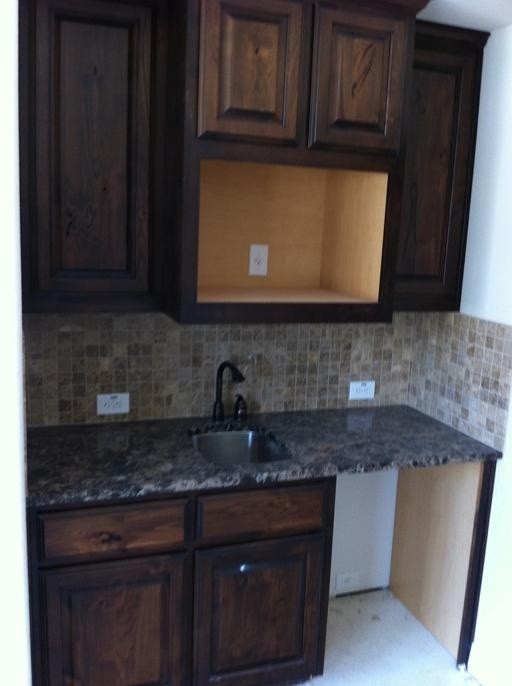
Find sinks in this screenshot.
[192,431,255,465]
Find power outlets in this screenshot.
[96,392,131,415]
[248,244,269,277]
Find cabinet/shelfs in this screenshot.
[189,481,325,686]
[157,0,412,150]
[157,142,398,324]
[394,40,481,304]
[32,0,156,304]
[38,494,192,686]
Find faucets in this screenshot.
[214,360,244,421]
